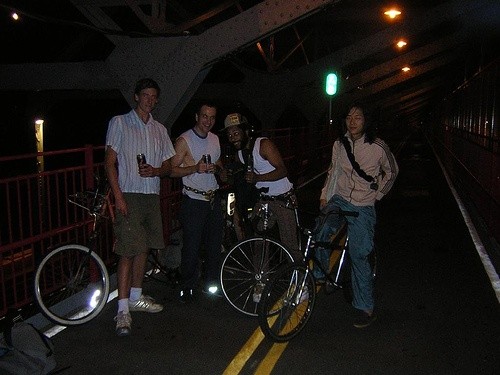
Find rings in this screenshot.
[247,178,249,180]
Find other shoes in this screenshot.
[201,281,224,297]
[179,287,193,304]
[305,277,326,285]
[352,309,377,328]
[253,283,270,303]
[300,290,309,304]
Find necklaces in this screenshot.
[352,136,356,140]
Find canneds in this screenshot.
[203,154,212,174]
[246,166,252,173]
[137,153,146,169]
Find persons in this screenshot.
[314,102,400,328]
[220,113,306,265]
[105,78,175,336]
[168,102,225,306]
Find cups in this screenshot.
[246,159,253,172]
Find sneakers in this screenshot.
[128,295,163,313]
[114,310,132,336]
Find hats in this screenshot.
[225,113,243,128]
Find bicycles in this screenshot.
[32,176,183,328]
[215,192,313,319]
[257,203,378,344]
